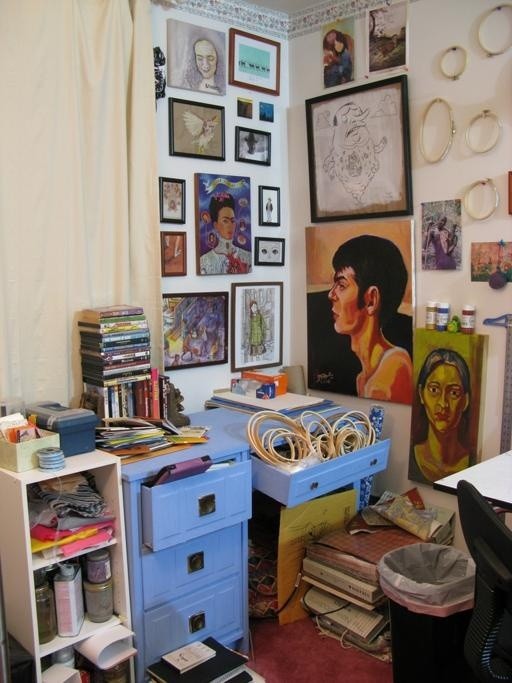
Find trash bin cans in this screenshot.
[377,544,478,682]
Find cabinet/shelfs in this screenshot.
[0,450,135,683]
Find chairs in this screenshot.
[456,479,512,683]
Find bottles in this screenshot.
[32,580,58,644]
[53,562,85,637]
[50,646,76,671]
[425,301,477,334]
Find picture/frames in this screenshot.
[305,74,413,224]
[160,290,229,372]
[254,237,285,266]
[159,230,187,278]
[234,126,272,166]
[158,177,187,224]
[231,281,283,372]
[228,27,283,97]
[167,97,226,161]
[258,185,281,226]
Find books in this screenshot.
[78,304,171,426]
[146,637,253,683]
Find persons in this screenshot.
[249,301,268,356]
[328,234,414,404]
[182,29,225,94]
[409,347,476,486]
[323,31,353,88]
[200,192,252,273]
[265,198,274,222]
[422,216,458,269]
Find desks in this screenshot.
[119,392,390,683]
[433,449,512,511]
